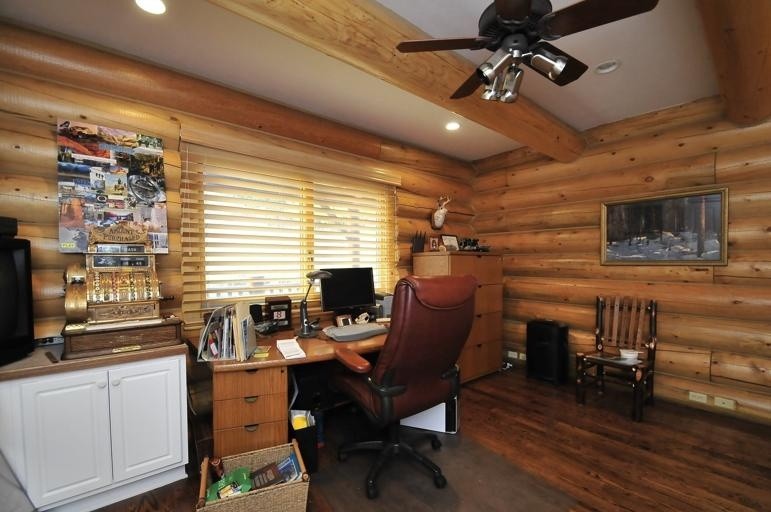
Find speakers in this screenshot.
[1,217,34,367]
[266,296,292,332]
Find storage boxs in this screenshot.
[412,251,503,385]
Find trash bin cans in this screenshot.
[289,422,318,472]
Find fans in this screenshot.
[396,0,659,100]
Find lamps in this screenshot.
[476,1,569,103]
[63,262,87,322]
[293,270,331,340]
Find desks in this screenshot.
[187,316,389,466]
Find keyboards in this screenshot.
[327,323,389,341]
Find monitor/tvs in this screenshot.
[319,267,376,312]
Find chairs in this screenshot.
[327,273,477,499]
[576,295,658,422]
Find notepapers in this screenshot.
[254,352,270,358]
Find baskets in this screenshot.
[195,439,310,512]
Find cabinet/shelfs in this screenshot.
[0,341,189,512]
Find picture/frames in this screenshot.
[600,185,728,266]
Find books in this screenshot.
[254,346,271,358]
